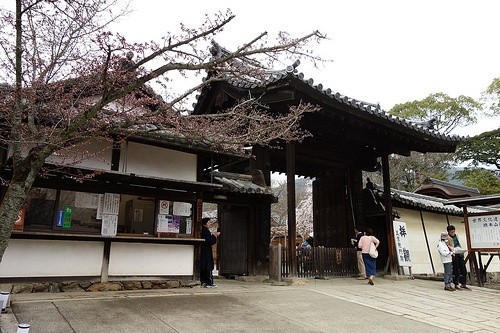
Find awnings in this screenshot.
[6,152,223,195]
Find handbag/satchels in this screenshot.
[369,241,379,259]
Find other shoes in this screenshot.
[454,286,460,290]
[368,277,374,285]
[444,287,452,290]
[357,277,365,280]
[200,282,217,288]
[460,286,469,290]
[450,286,456,291]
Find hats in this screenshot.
[440,232,449,240]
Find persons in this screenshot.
[447,225,470,290]
[437,233,455,291]
[200,217,220,287]
[351,225,368,279]
[358,226,380,285]
[296,234,313,275]
